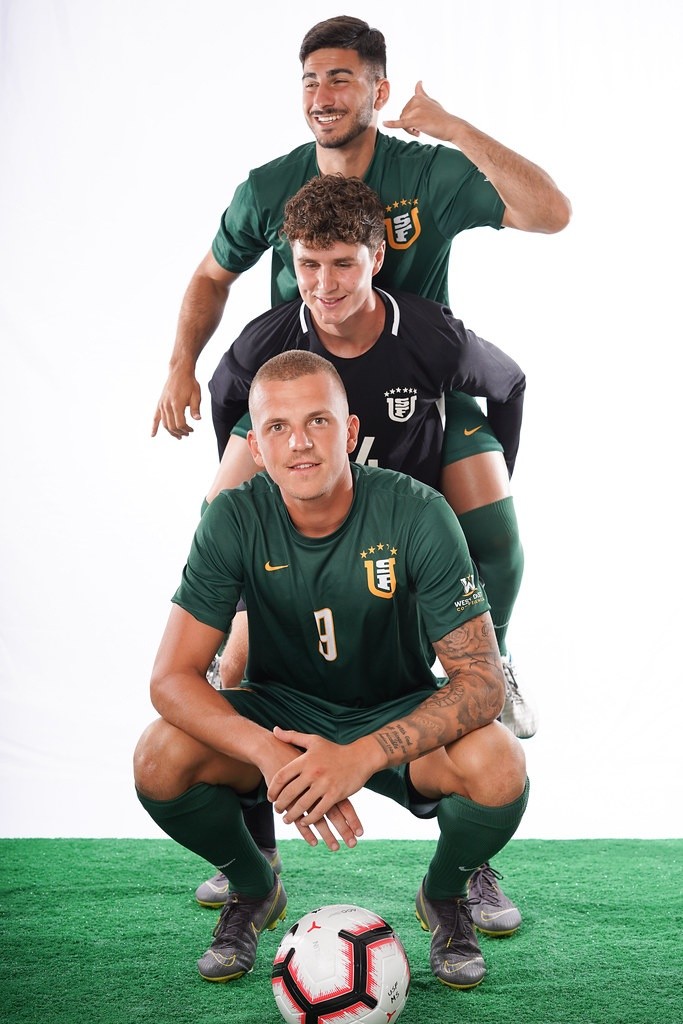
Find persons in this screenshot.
[193,170,525,937]
[135,350,531,990]
[150,14,574,738]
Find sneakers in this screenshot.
[195,847,282,907]
[497,653,538,739]
[466,859,521,937]
[197,870,286,981]
[414,872,486,990]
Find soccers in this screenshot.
[272,905,409,1024]
